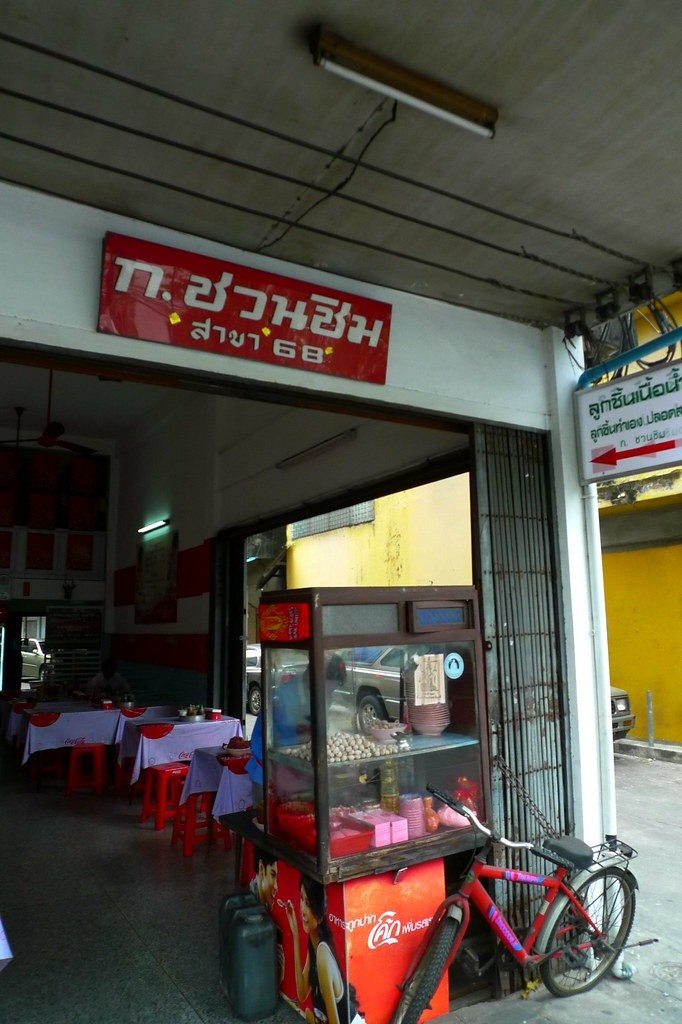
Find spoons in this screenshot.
[277,899,290,908]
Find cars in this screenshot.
[332,642,636,754]
[18,635,53,682]
[246,641,311,716]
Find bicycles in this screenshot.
[385,785,659,1024]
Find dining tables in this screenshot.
[6,697,96,765]
[21,701,185,765]
[126,713,244,785]
[192,746,253,828]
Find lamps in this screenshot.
[136,519,170,534]
[307,28,499,140]
[275,428,357,470]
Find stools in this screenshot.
[65,743,108,800]
[114,743,143,801]
[171,781,232,856]
[139,761,190,831]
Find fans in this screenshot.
[0,361,100,458]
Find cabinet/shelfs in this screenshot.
[255,587,494,883]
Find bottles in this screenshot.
[378,757,402,815]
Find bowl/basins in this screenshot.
[118,700,136,707]
[403,668,452,735]
[180,713,208,722]
[370,722,408,743]
[223,744,252,756]
